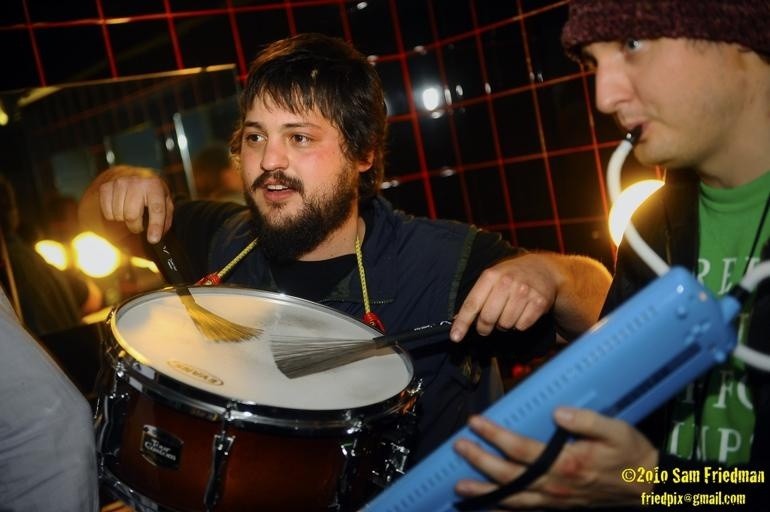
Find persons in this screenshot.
[452,3,769,512]
[77,34,614,512]
[1,172,82,337]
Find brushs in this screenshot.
[271,316,475,379]
[143,208,264,344]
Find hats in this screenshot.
[562,1,768,59]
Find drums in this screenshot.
[94,282,426,510]
